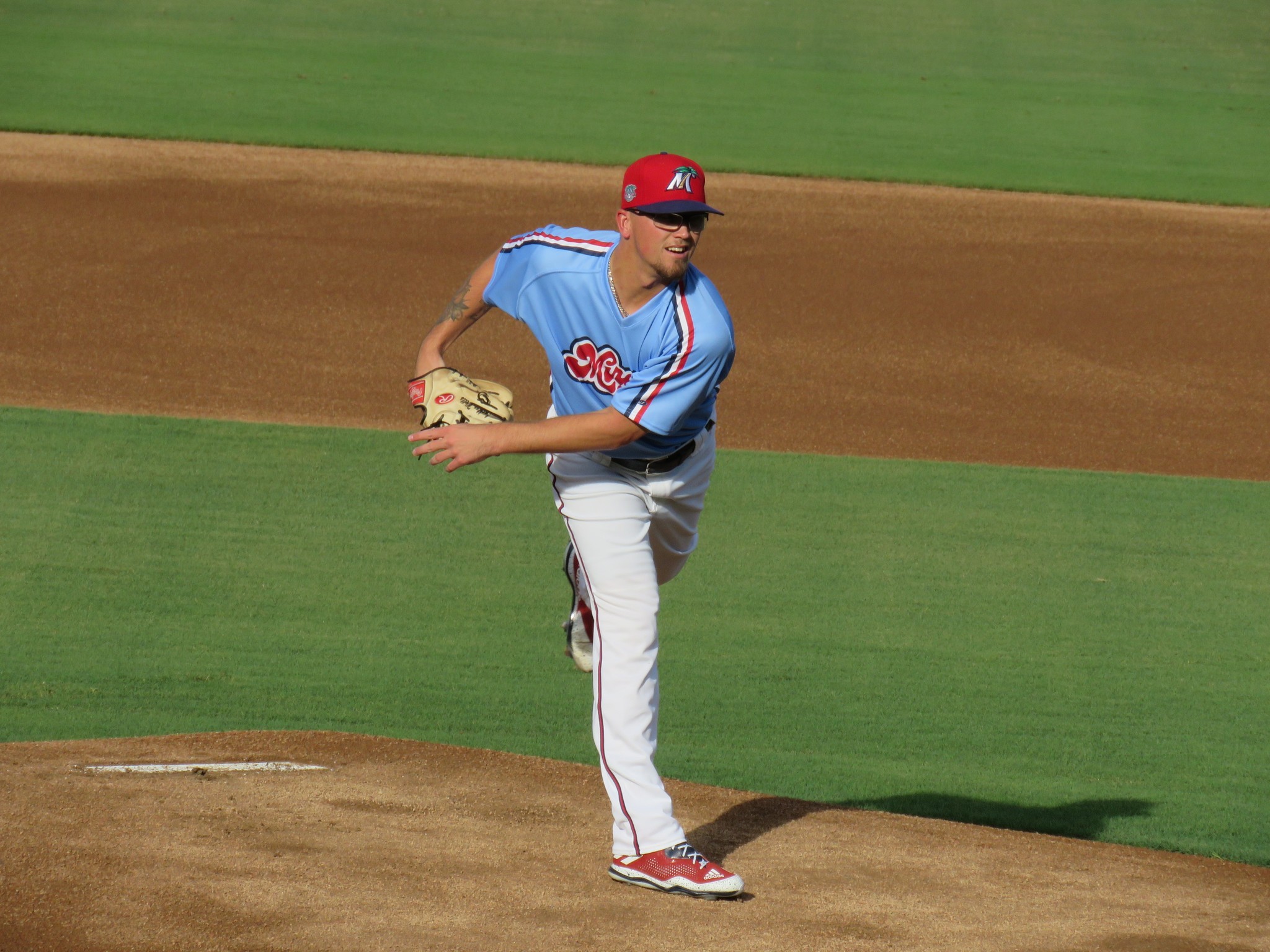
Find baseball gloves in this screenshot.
[408,366,515,441]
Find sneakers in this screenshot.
[561,540,595,673]
[608,842,744,900]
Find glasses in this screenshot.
[624,207,709,233]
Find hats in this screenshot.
[621,152,725,216]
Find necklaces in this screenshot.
[608,255,630,318]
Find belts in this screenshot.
[611,419,714,475]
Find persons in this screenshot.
[408,152,745,900]
